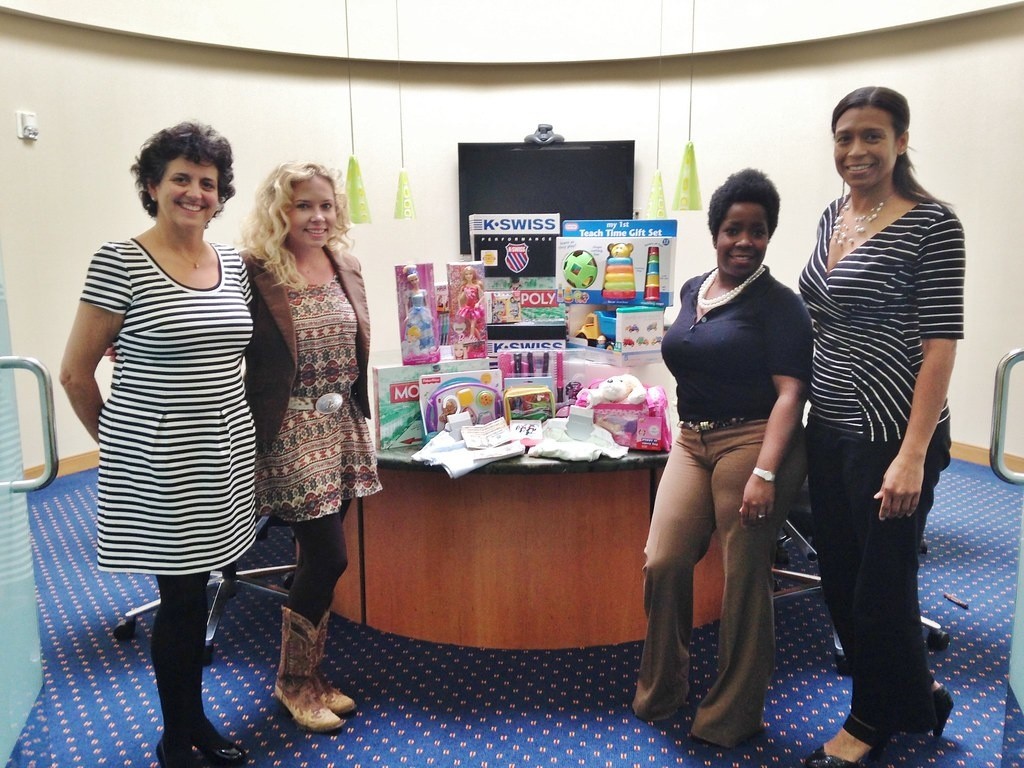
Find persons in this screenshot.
[59,123,259,768]
[402,265,438,353]
[458,267,483,344]
[799,86,966,768]
[633,170,814,746]
[105,161,385,730]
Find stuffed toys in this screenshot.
[581,374,647,408]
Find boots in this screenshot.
[275,605,357,733]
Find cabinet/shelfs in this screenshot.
[270,426,728,648]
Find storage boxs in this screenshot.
[578,377,671,453]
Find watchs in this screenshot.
[753,467,776,482]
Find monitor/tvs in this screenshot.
[458,140,635,254]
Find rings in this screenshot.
[757,515,767,519]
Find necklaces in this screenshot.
[833,191,896,245]
[155,227,205,269]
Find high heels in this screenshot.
[805,733,892,768]
[156,719,245,768]
[896,683,954,736]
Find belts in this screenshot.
[288,390,350,415]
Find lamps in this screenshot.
[343,0,704,223]
